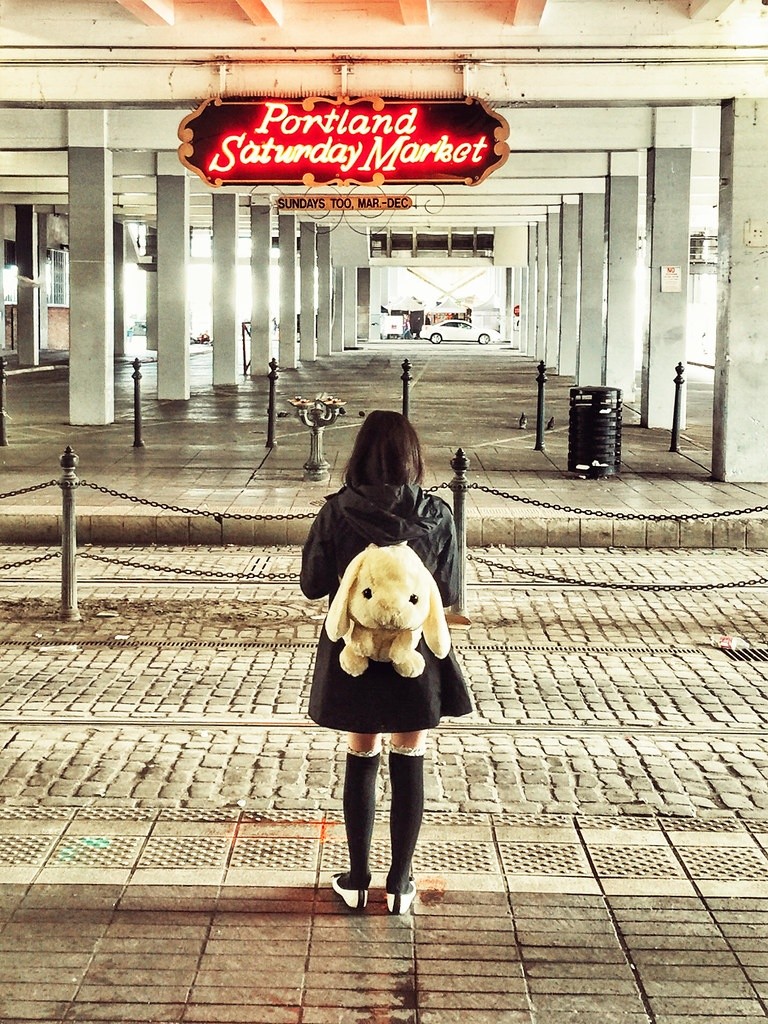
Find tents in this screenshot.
[431,297,466,323]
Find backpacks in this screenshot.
[326,538,453,679]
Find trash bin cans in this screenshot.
[565,385,624,481]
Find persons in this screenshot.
[299,410,474,913]
[124,315,278,343]
[402,315,471,339]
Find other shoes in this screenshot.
[333,873,370,910]
[387,878,416,915]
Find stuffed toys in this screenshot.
[324,540,451,678]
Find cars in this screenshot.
[419,321,502,345]
